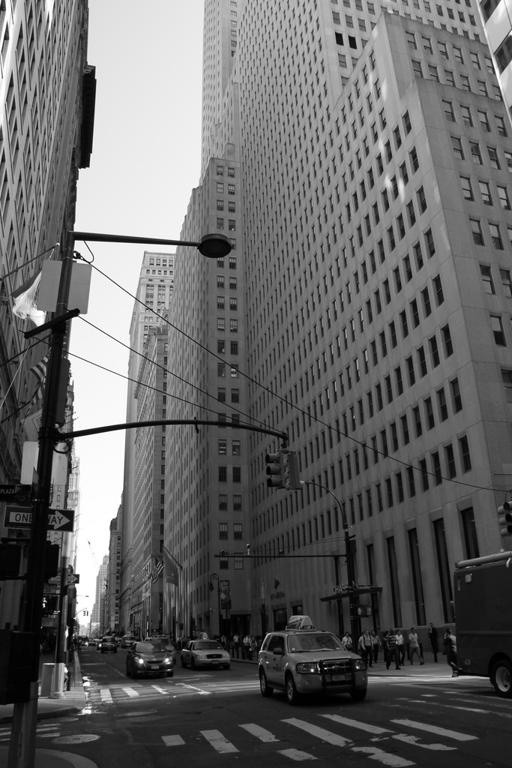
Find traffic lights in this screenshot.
[497,502,512,537]
[265,451,284,488]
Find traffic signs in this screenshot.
[4,506,74,533]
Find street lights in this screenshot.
[11,230,232,763]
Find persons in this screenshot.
[217,629,270,660]
[440,627,463,677]
[426,622,441,663]
[342,626,424,670]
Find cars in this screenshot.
[78,635,134,654]
[126,642,174,680]
[180,640,230,671]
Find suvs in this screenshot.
[257,630,367,704]
[144,634,177,665]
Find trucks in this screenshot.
[450,552,511,697]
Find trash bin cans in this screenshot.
[41,662,56,696]
